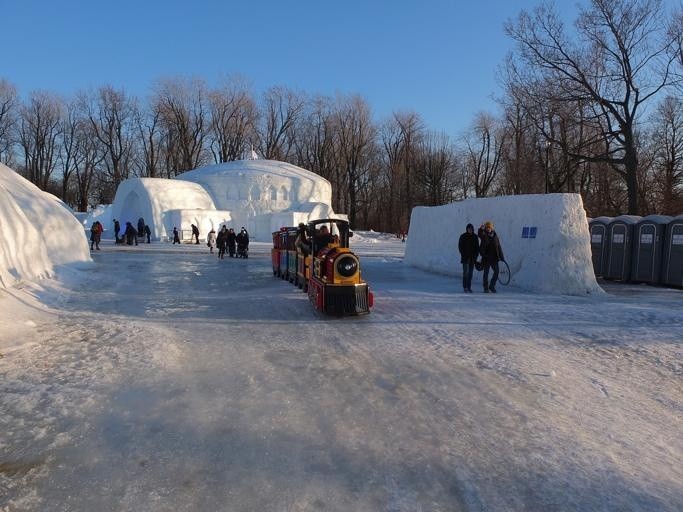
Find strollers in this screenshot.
[236,244,248,259]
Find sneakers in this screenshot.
[464,287,496,294]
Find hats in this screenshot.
[486,222,493,228]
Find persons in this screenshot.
[458,223,480,293]
[294,220,350,257]
[478,221,504,292]
[90,217,250,259]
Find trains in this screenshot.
[272,218,374,318]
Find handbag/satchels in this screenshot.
[475,262,483,271]
